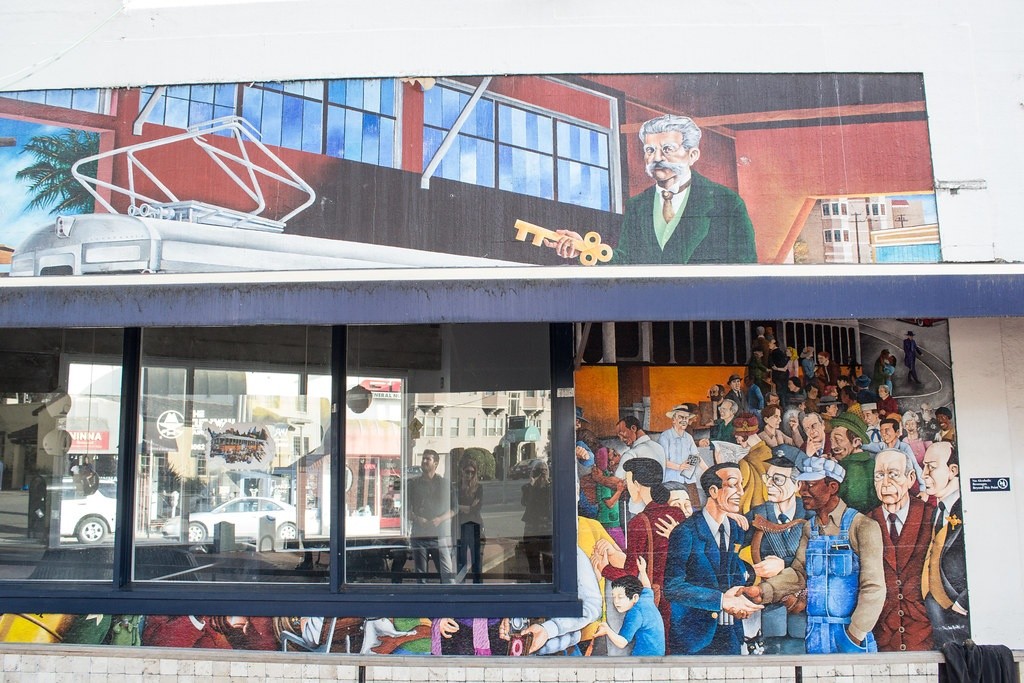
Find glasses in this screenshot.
[464,470,475,474]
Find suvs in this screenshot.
[59,476,116,545]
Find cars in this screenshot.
[159,496,320,542]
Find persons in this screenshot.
[520,461,554,586]
[163,488,179,517]
[450,460,484,584]
[247,483,259,507]
[71,463,81,483]
[406,449,453,583]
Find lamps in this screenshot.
[72,329,100,496]
[345,325,375,413]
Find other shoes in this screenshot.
[295,562,314,570]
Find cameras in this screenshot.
[532,470,542,478]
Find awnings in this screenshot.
[509,426,541,442]
